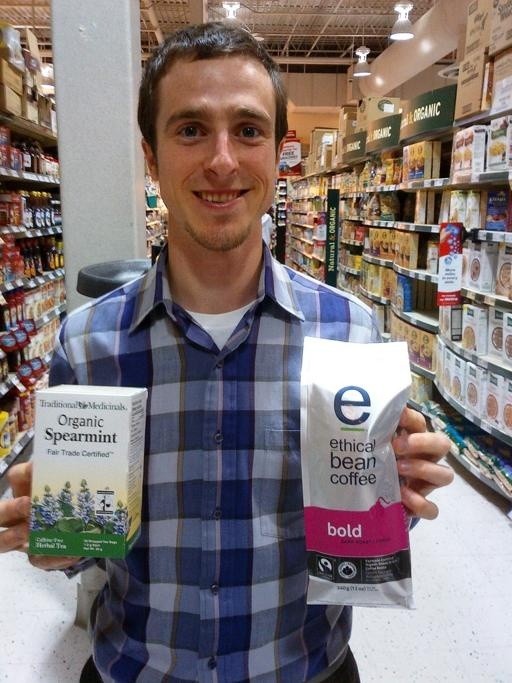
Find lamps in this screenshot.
[251,32,264,41]
[222,1,241,20]
[389,2,415,40]
[353,19,372,78]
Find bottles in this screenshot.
[0,290,34,454]
[0,141,63,284]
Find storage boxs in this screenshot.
[0,26,57,138]
[454,1,512,124]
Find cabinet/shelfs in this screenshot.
[145,174,169,259]
[275,177,286,263]
[0,109,67,478]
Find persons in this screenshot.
[0,20,454,683]
